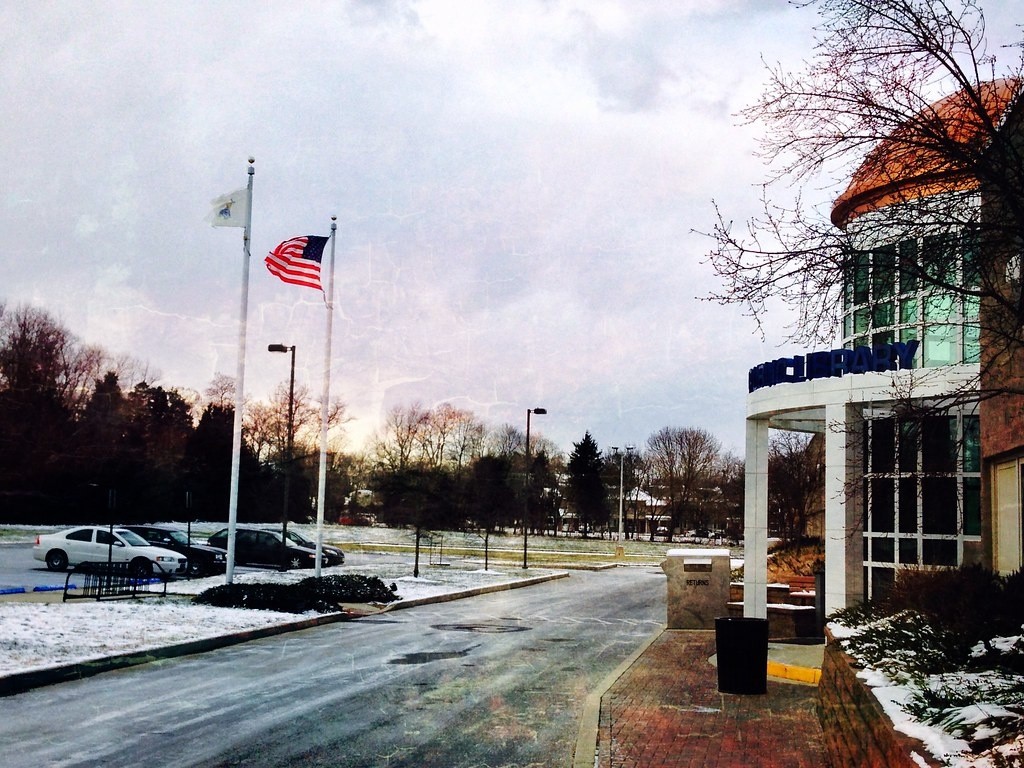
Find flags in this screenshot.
[264,235,329,290]
[205,188,252,227]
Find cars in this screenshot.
[120,525,236,578]
[687,529,712,537]
[562,524,591,532]
[33,527,188,580]
[208,527,329,573]
[258,528,345,568]
[656,526,669,536]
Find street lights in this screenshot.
[612,445,634,541]
[267,344,297,573]
[522,407,547,568]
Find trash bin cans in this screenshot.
[715,617,770,695]
[661,548,729,629]
